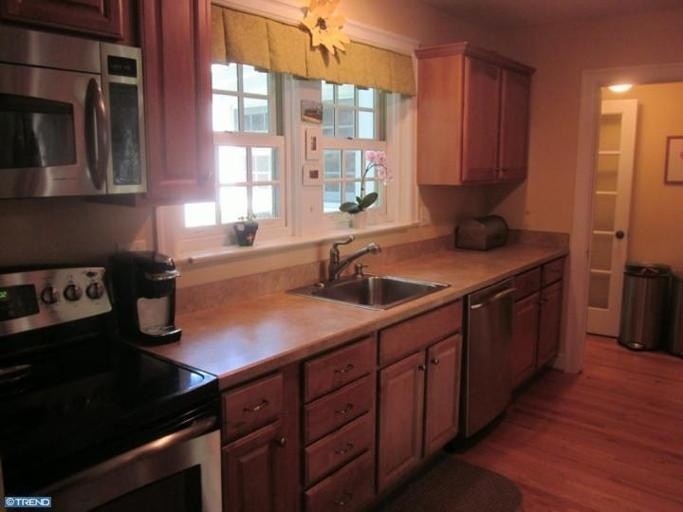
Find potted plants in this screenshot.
[231,212,258,246]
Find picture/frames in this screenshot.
[662,135,682,186]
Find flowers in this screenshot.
[339,151,397,220]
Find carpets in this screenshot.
[374,457,521,509]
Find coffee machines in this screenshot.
[106,246,183,345]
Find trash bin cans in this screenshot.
[618,263,683,355]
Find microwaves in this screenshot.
[0,25,145,196]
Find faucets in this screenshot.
[328,235,381,280]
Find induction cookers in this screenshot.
[1,310,222,482]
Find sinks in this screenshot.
[286,273,451,311]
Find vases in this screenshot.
[348,210,366,232]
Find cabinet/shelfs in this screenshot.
[301,333,376,510]
[414,42,536,187]
[130,1,215,206]
[219,360,301,510]
[511,254,564,388]
[1,1,121,40]
[378,294,462,498]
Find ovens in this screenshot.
[459,276,516,438]
[12,427,223,511]
